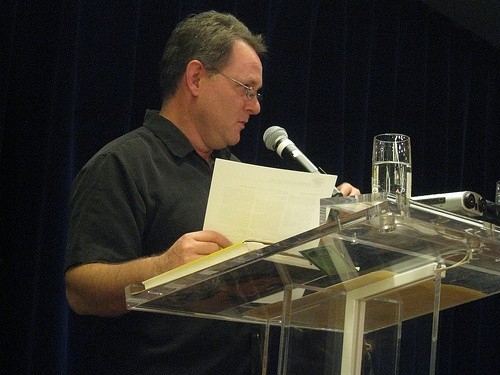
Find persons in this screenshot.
[59,10,363,375]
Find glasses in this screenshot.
[212,66,262,99]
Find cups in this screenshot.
[371,133,411,230]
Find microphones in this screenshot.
[263,126,343,197]
